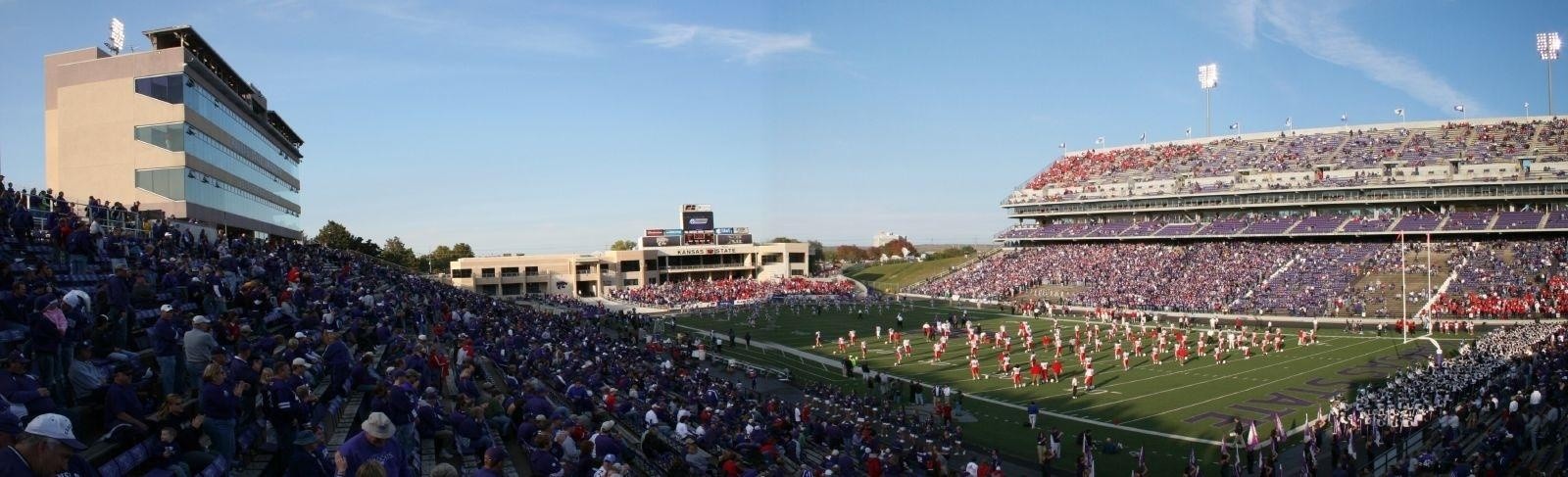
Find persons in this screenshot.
[996,118,1567,476]
[0,177,1001,476]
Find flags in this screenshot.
[1454,104,1466,114]
[1341,110,1349,121]
[1394,108,1406,118]
[1521,103,1530,113]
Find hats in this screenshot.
[0,227,983,477]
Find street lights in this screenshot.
[1198,63,1219,137]
[104,16,125,56]
[1536,32,1562,116]
